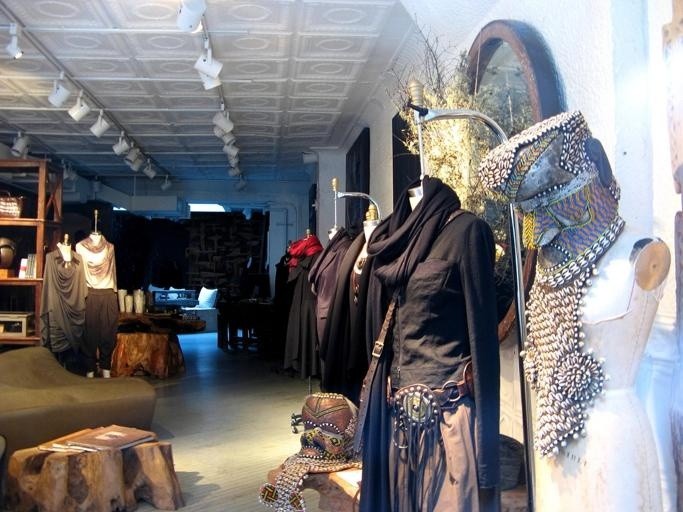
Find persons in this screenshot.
[478,110,677,512]
[39,242,88,373]
[201,257,270,352]
[74,231,119,379]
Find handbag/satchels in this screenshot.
[0,190,23,218]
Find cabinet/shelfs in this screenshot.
[0,158,63,350]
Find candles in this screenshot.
[116,288,145,314]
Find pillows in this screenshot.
[194,287,217,308]
[148,283,185,300]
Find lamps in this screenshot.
[48,67,172,193]
[193,40,248,193]
[7,132,30,158]
[177,1,208,34]
[5,22,24,63]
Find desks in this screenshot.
[4,421,186,512]
[108,313,207,382]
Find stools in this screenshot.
[0,343,157,504]
